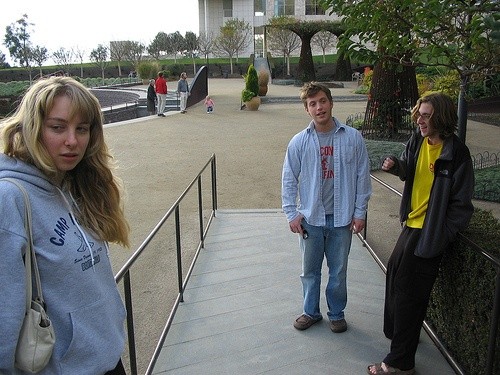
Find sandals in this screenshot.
[367,361,415,375]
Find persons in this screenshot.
[204,96,215,114]
[367,92,475,375]
[155,72,167,117]
[147,79,157,115]
[0,75,127,375]
[281,81,372,333]
[177,72,191,114]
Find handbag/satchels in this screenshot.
[0,177,56,375]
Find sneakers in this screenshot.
[293,314,323,330]
[329,319,347,333]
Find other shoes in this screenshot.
[207,112,209,113]
[158,113,166,117]
[210,111,213,113]
[180,110,187,114]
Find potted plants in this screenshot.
[136,61,177,85]
[241,64,261,111]
[258,64,270,96]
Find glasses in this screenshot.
[415,113,431,120]
[300,224,309,239]
